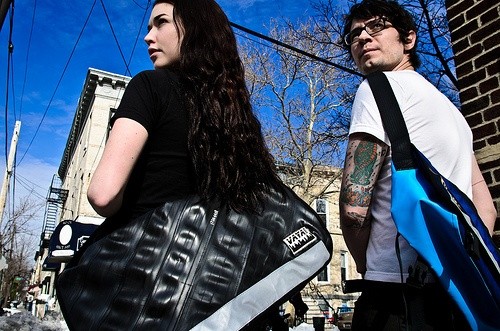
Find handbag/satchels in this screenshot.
[56,164,334,331]
[391,143,500,331]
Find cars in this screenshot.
[337,312,354,331]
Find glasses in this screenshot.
[344,16,391,45]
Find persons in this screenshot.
[52,0,334,331]
[339,0,497,331]
